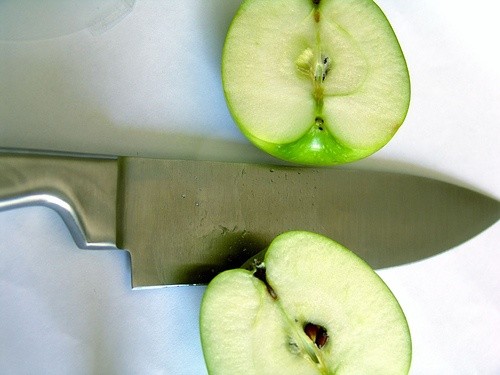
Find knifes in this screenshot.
[1,147,500,291]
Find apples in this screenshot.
[197,229,413,375]
[220,0,412,168]
